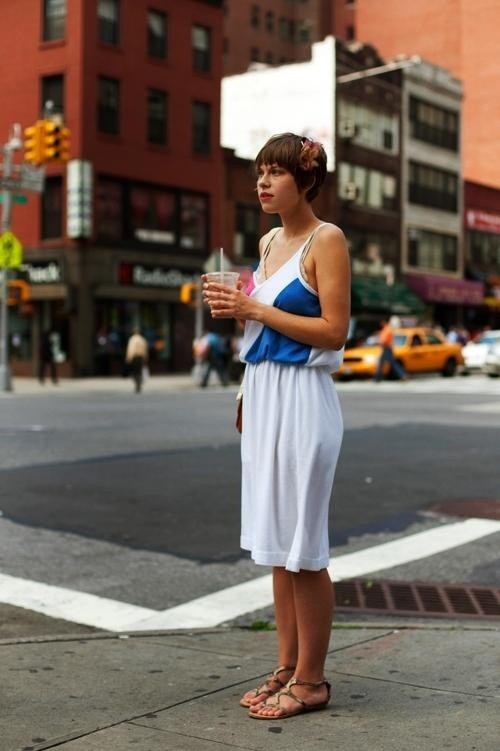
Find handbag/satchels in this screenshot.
[233,378,245,435]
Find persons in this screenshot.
[38,328,66,382]
[201,132,352,720]
[192,327,228,389]
[124,324,152,395]
[370,317,411,381]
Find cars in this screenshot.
[333,324,467,381]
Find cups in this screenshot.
[206,272,240,318]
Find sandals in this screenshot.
[239,665,296,708]
[249,676,332,722]
